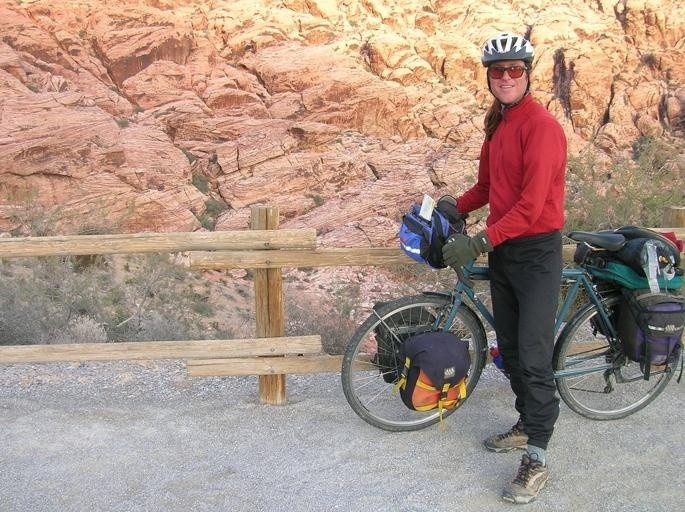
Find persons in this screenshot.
[441,31,565,504]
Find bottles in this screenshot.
[490,348,505,369]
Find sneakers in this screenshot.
[485,418,529,453]
[502,456,548,503]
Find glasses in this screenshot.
[488,66,528,79]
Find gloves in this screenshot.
[442,230,492,270]
[428,202,465,269]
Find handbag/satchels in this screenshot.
[401,204,451,265]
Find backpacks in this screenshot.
[574,226,685,366]
[373,301,472,412]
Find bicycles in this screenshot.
[341,203,685,432]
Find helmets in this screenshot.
[481,32,535,68]
